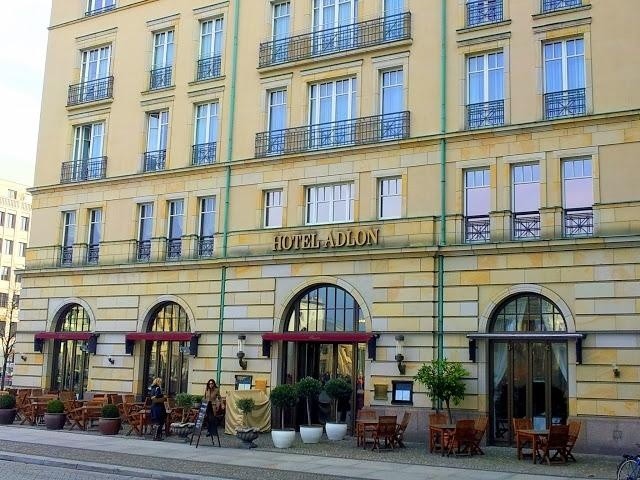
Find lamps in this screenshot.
[20,351,27,362]
[236,334,248,370]
[611,361,622,378]
[106,355,116,365]
[394,335,407,374]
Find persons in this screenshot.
[150,378,167,441]
[204,378,225,437]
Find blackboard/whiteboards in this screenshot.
[193,399,209,436]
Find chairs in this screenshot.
[371,415,398,453]
[358,409,381,449]
[386,411,413,449]
[428,412,582,466]
[1,386,226,437]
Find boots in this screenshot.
[154,426,164,441]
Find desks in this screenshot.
[224,388,271,435]
[356,418,402,449]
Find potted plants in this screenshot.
[324,377,352,441]
[269,384,299,448]
[295,376,325,445]
[235,397,261,449]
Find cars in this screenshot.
[0,365,13,387]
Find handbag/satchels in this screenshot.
[151,397,164,403]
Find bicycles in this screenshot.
[615,452,640,480]
